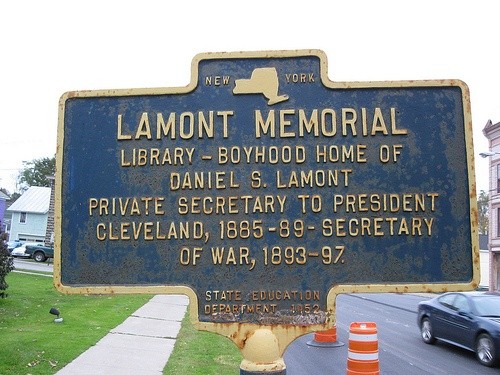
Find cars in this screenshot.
[417,290,500,367]
[5,239,55,261]
